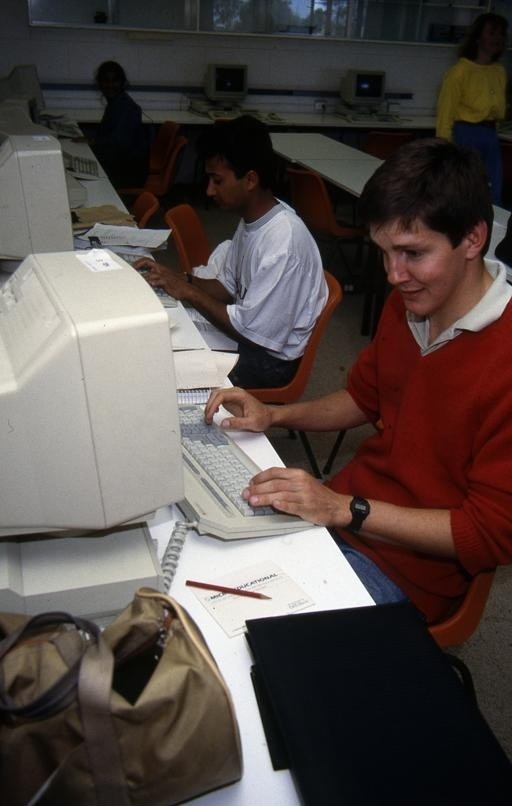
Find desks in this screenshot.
[300,158,511,278]
[268,131,384,162]
[1,112,378,805]
[44,109,435,127]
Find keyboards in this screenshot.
[174,404,317,541]
[208,110,281,121]
[116,243,177,310]
[61,150,99,180]
[346,111,400,123]
[49,119,84,139]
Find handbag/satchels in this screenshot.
[2,586,243,805]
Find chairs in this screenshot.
[130,190,161,229]
[109,135,188,197]
[165,203,210,274]
[246,270,344,478]
[286,167,365,264]
[427,569,500,649]
[149,119,181,173]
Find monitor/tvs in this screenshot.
[339,69,386,115]
[2,249,187,540]
[0,135,75,273]
[204,62,248,107]
[0,64,46,111]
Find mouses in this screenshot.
[268,111,281,121]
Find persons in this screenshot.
[133,116,330,392]
[209,138,512,631]
[70,60,152,191]
[435,15,510,202]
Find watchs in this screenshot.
[348,492,370,533]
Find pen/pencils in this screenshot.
[186,580,271,599]
